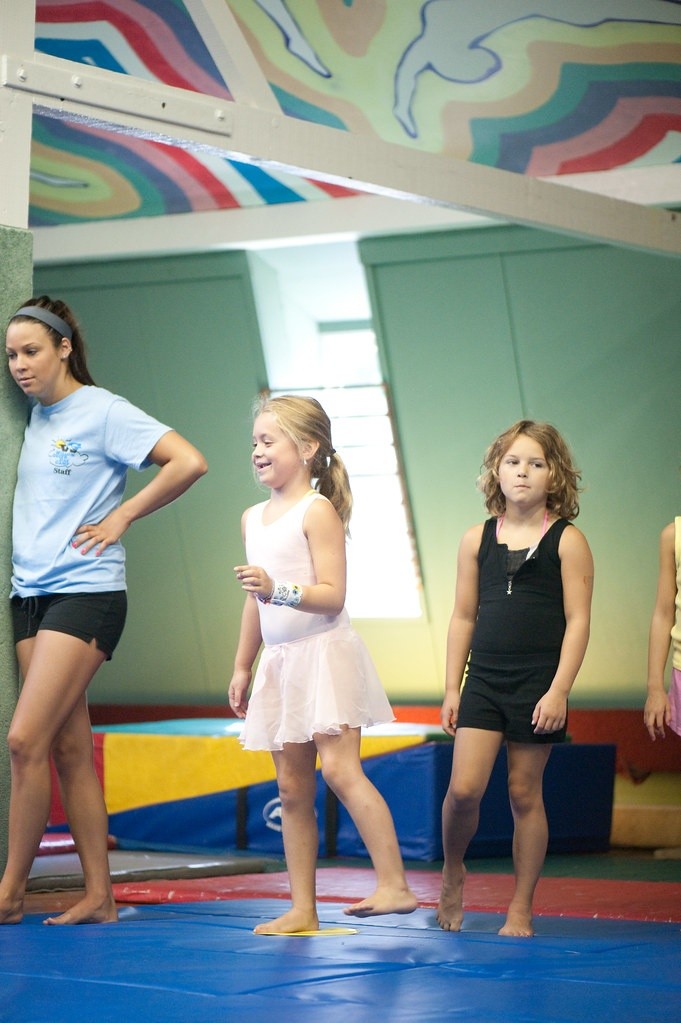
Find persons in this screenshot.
[226,396,419,935]
[437,421,595,938]
[643,512,681,741]
[0,295,207,925]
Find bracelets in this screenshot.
[259,578,303,607]
